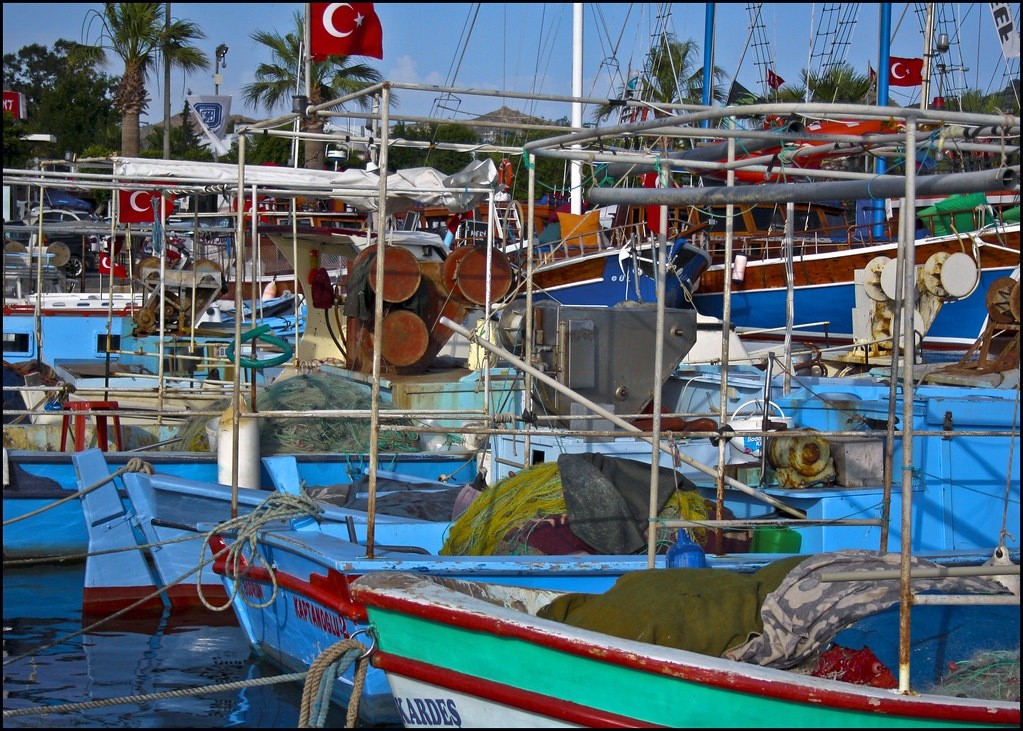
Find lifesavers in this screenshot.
[498,158,513,193]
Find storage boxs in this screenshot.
[826,435,884,487]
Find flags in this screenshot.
[98,251,128,279]
[311,2,383,59]
[232,197,269,223]
[888,57,924,87]
[767,69,785,90]
[116,179,176,223]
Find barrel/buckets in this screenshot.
[204,417,222,452]
[726,399,793,456]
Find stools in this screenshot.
[60,401,122,452]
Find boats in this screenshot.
[3,2,1021,728]
